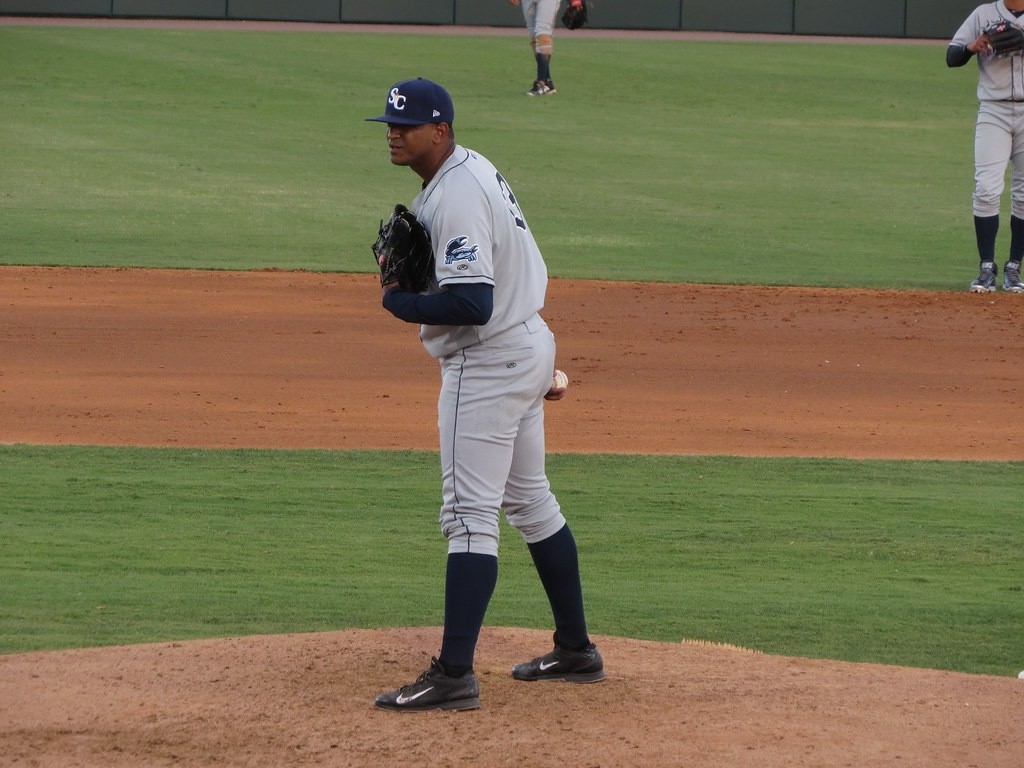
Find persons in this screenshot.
[947,0,1024,294]
[365,76,606,711]
[507,0,588,97]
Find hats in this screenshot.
[365,77,455,125]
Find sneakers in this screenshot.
[526,80,556,96]
[512,643,606,684]
[969,259,998,293]
[1001,259,1024,293]
[375,656,480,712]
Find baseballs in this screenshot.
[548,369,568,395]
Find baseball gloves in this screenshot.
[372,203,431,298]
[983,17,1024,57]
[562,1,586,29]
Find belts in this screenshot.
[470,313,545,345]
